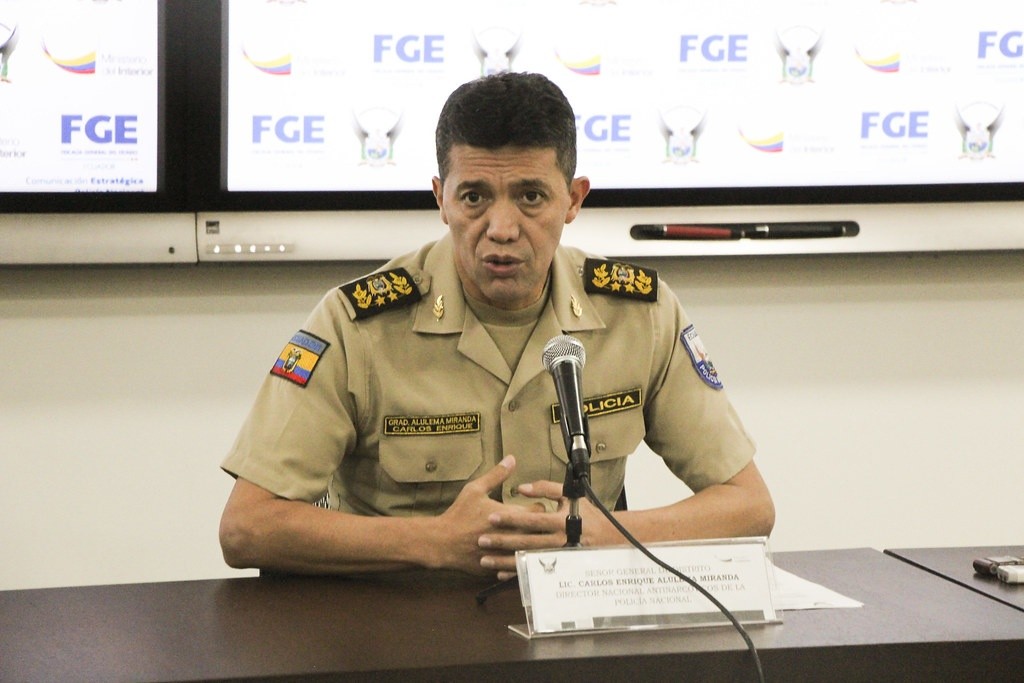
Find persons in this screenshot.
[218,73,777,579]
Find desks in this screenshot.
[0,544,1024,683]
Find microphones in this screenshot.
[542,334,591,479]
